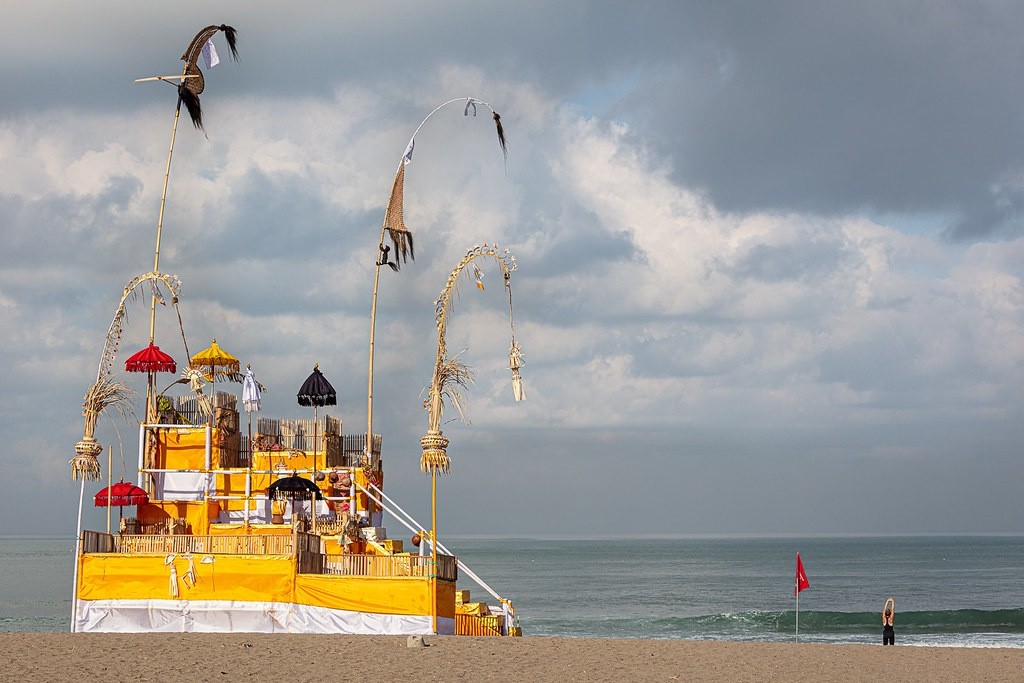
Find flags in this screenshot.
[793,553,810,597]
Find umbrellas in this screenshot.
[264,469,320,512]
[297,361,337,423]
[242,363,260,423]
[189,338,240,381]
[123,342,176,378]
[93,476,148,522]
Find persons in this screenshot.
[882,598,896,646]
